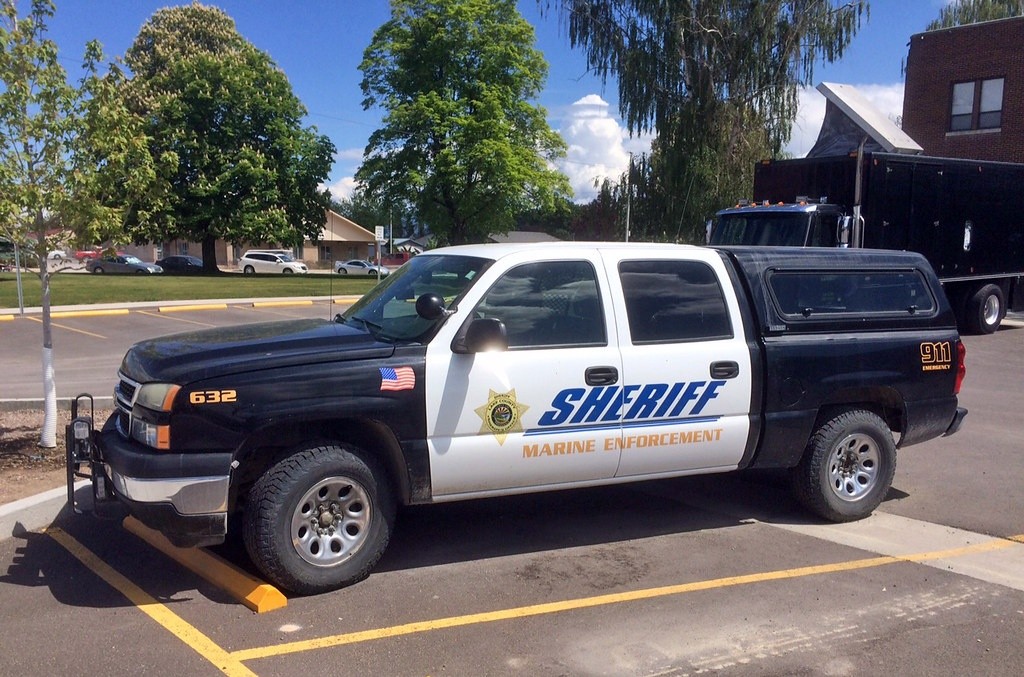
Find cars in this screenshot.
[155,254,220,273]
[236,249,309,275]
[84,253,164,275]
[74,244,127,264]
[46,245,67,260]
[329,262,389,276]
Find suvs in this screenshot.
[64,239,970,598]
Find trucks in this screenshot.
[704,150,1024,335]
